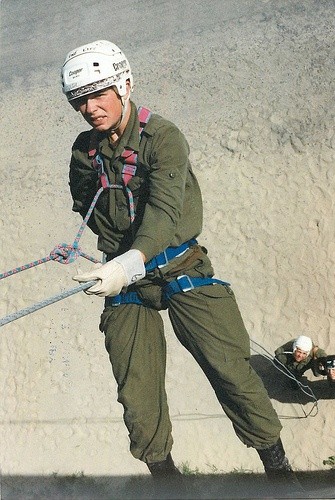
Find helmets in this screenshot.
[61,40,134,113]
[325,355,335,369]
[293,336,312,359]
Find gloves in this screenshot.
[73,248,146,297]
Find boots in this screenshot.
[147,452,185,499]
[256,437,309,496]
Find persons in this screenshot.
[274,336,335,392]
[60,38,306,498]
